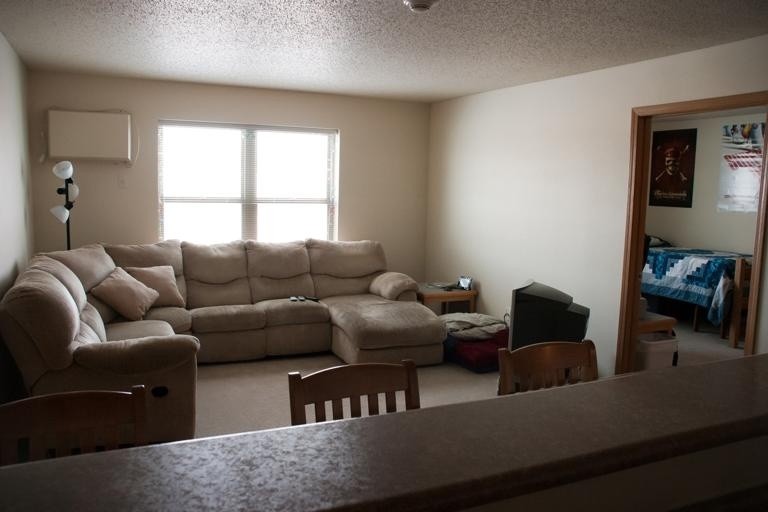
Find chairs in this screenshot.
[288,359,420,427]
[499,340,598,394]
[0,384,147,466]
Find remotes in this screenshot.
[298,295,306,301]
[305,296,320,302]
[289,296,298,301]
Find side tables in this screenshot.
[417,282,478,316]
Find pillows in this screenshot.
[90,267,160,320]
[123,266,185,307]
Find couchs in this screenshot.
[0,234,444,443]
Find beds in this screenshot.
[641,236,754,348]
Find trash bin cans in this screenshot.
[636,333,680,370]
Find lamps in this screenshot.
[49,162,78,250]
[403,0,440,14]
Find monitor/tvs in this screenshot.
[508,280,592,352]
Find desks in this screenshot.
[638,312,679,368]
